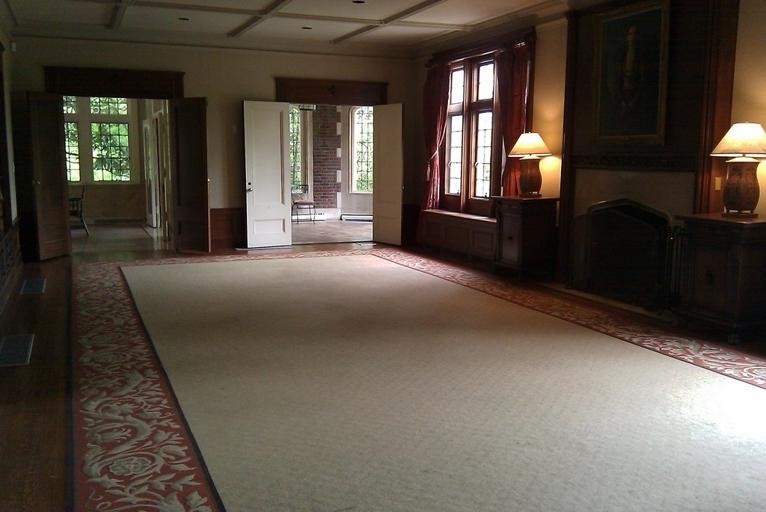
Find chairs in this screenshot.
[291,184,317,224]
[67,184,91,237]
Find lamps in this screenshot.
[507,131,553,196]
[710,121,766,217]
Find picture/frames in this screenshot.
[592,0,670,146]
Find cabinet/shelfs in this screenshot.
[490,195,559,280]
[674,212,766,345]
[419,209,498,262]
